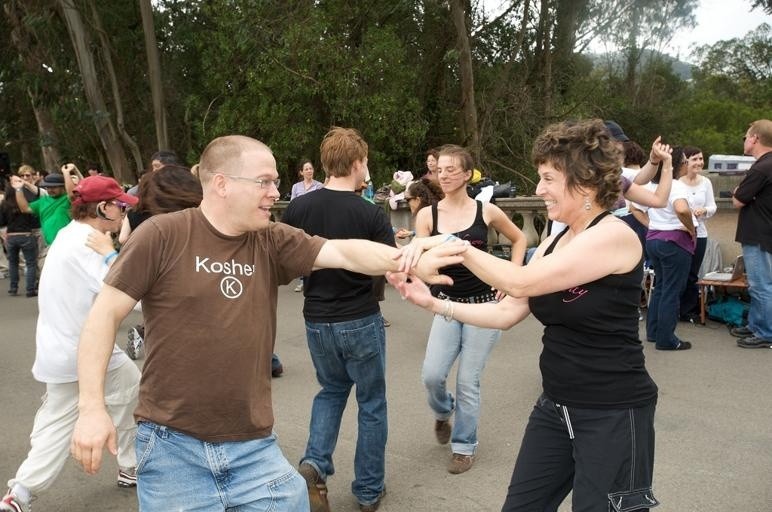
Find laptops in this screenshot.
[703,255,744,282]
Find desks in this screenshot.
[695,271,749,326]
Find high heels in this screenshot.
[294,284,303,293]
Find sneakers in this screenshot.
[730,326,752,337]
[359,484,386,512]
[117,471,137,487]
[271,363,282,376]
[126,327,142,360]
[0,493,31,512]
[435,419,451,444]
[299,464,330,512]
[448,453,475,474]
[8,290,17,296]
[737,334,772,348]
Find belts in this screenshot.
[437,293,496,304]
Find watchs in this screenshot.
[14,188,24,191]
[648,156,660,166]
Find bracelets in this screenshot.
[104,252,119,265]
[661,167,674,171]
[443,299,454,323]
[453,239,471,257]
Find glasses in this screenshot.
[105,200,128,212]
[224,175,280,190]
[407,197,416,202]
[682,160,689,164]
[21,174,30,177]
[742,135,757,141]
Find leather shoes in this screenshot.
[656,340,691,350]
[26,292,38,297]
[646,334,657,342]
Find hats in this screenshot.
[39,174,65,186]
[603,120,630,141]
[72,176,139,205]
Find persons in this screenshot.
[0,174,141,512]
[355,182,391,327]
[117,204,152,360]
[68,133,469,511]
[130,166,282,377]
[288,160,325,293]
[629,145,697,351]
[603,120,674,185]
[10,164,76,247]
[397,179,445,241]
[423,150,439,179]
[730,118,772,348]
[126,170,148,198]
[0,178,8,250]
[150,150,180,173]
[36,170,49,182]
[83,167,101,178]
[16,164,42,289]
[625,138,652,321]
[677,144,718,328]
[0,179,39,297]
[282,125,398,510]
[381,119,660,511]
[611,144,674,210]
[412,142,527,475]
